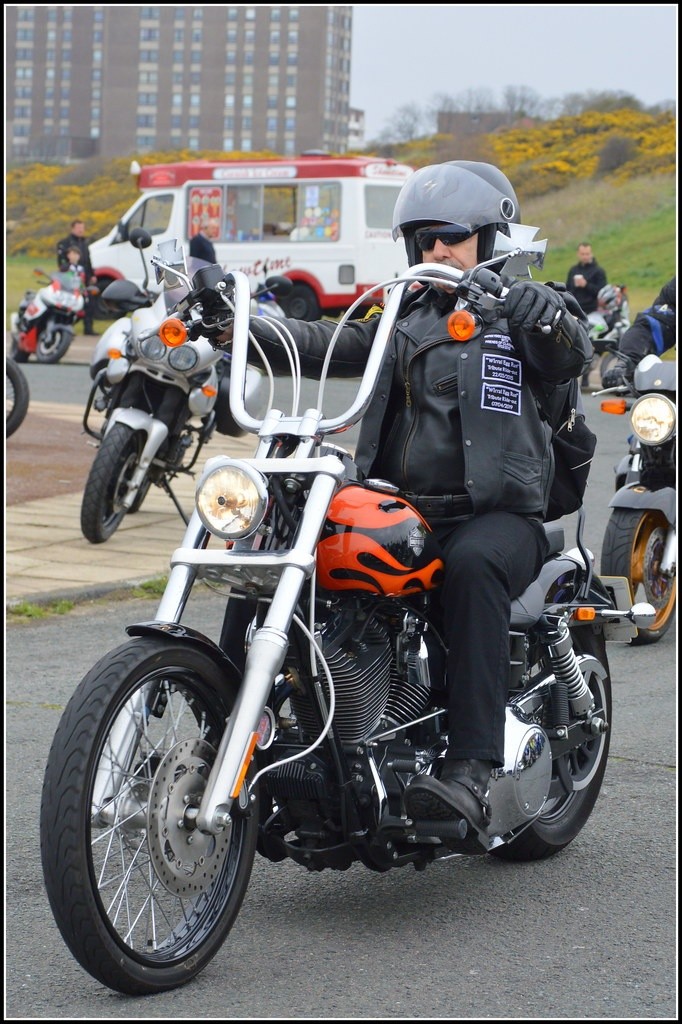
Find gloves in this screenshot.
[602,358,633,395]
[502,281,567,346]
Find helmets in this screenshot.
[597,283,627,311]
[400,162,520,286]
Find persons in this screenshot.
[55,220,101,336]
[204,158,597,859]
[566,240,607,313]
[190,217,220,266]
[601,275,676,398]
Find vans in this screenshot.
[80,150,415,323]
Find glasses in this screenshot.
[415,223,478,252]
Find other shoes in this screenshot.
[84,330,101,335]
[404,757,492,856]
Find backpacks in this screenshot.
[543,377,596,523]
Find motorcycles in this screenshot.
[70,227,292,546]
[586,337,680,649]
[6,267,85,365]
[575,283,635,389]
[37,231,657,999]
